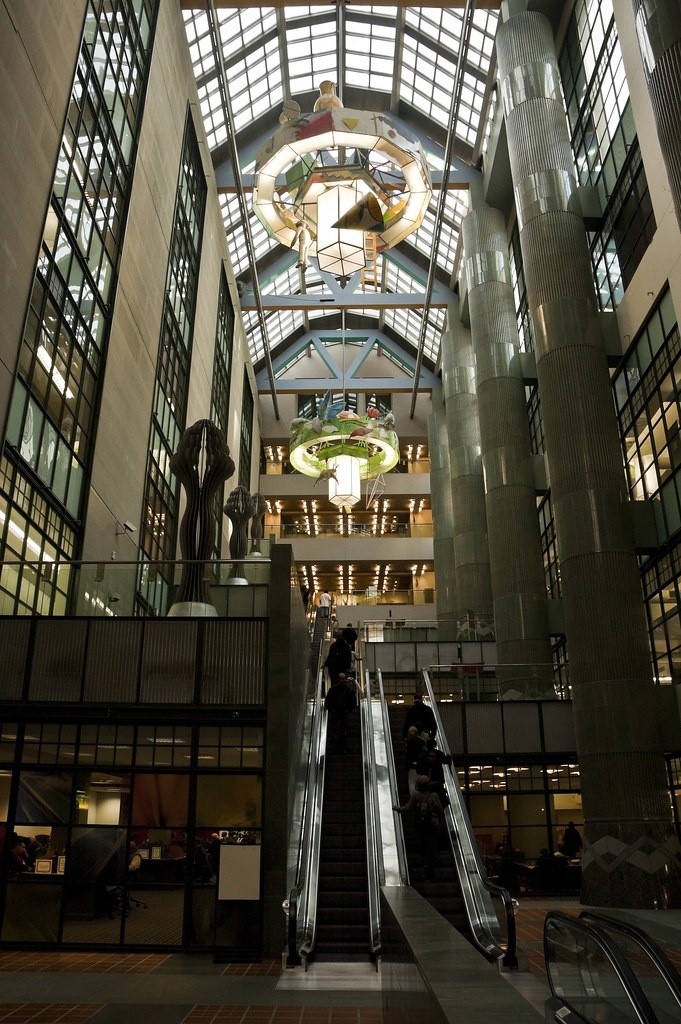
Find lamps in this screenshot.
[290,306,399,506]
[253,0,433,274]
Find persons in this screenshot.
[392,693,454,883]
[6,840,28,871]
[495,820,583,889]
[128,841,142,880]
[319,590,331,618]
[321,622,364,754]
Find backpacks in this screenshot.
[415,799,432,827]
